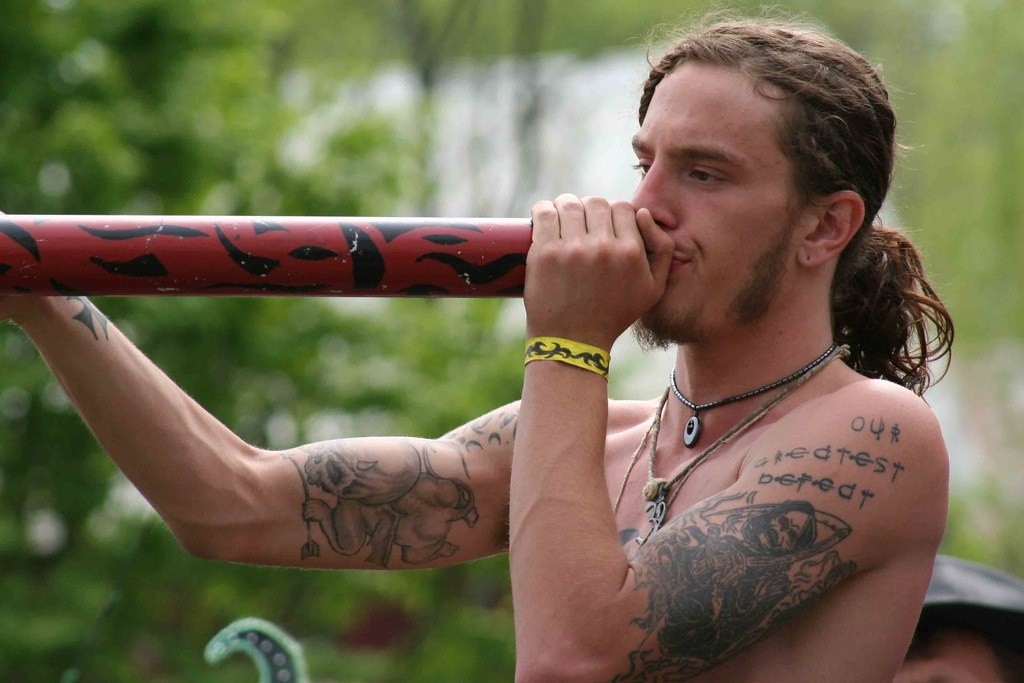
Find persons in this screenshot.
[1,16,949,683]
[895,554,1022,682]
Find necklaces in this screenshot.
[610,343,850,546]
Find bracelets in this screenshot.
[524,336,610,379]
